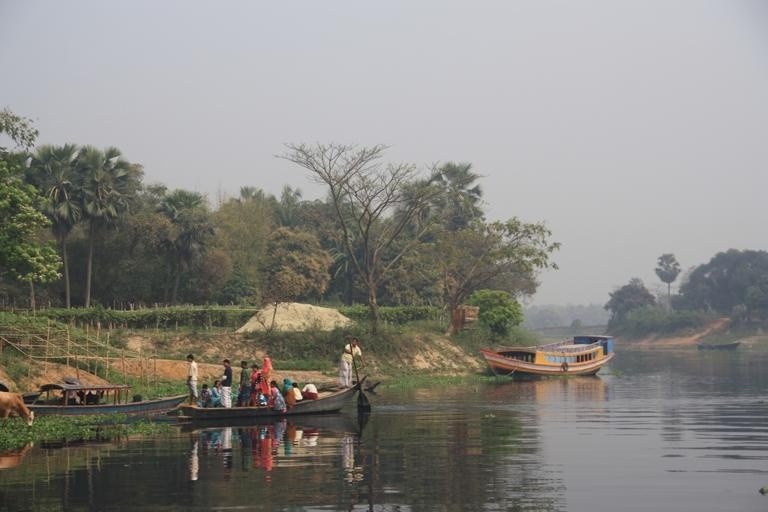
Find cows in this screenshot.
[0,439,34,470]
[0,389,37,431]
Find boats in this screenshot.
[38,432,143,451]
[178,375,368,417]
[482,375,611,403]
[479,335,616,379]
[179,413,361,435]
[697,342,740,350]
[16,383,191,419]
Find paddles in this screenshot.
[348,338,371,412]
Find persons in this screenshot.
[339,335,366,389]
[185,354,199,405]
[187,424,321,483]
[198,357,318,412]
[59,390,105,405]
[339,433,366,488]
